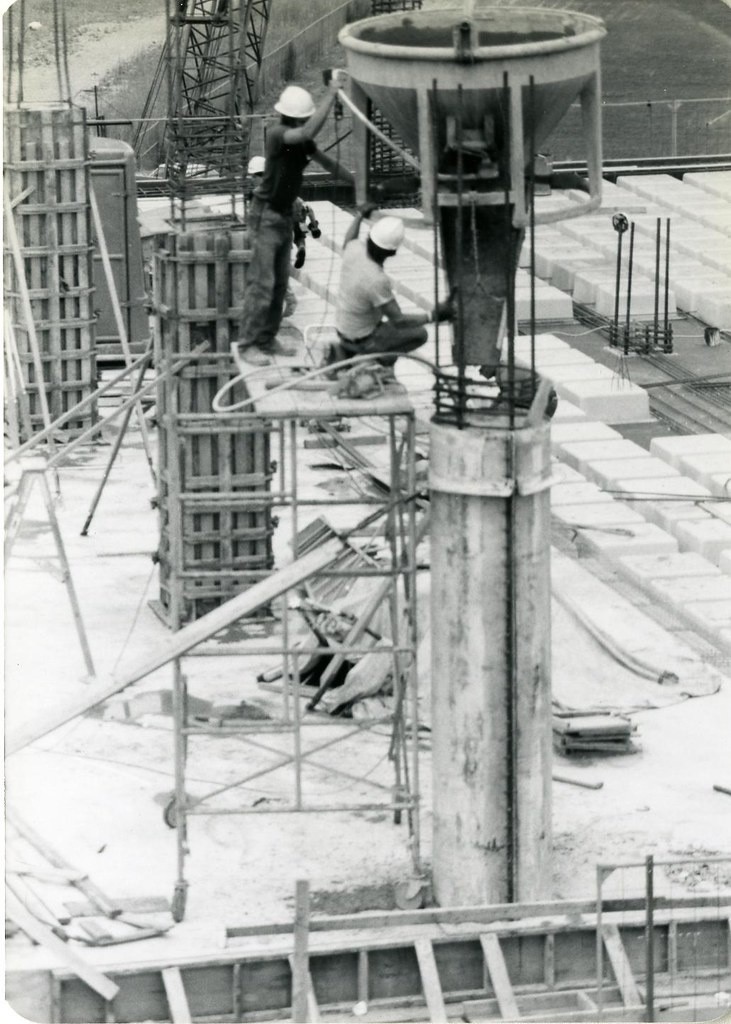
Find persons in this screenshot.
[247,156,321,317]
[333,201,454,386]
[237,79,343,366]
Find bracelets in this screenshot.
[427,312,433,322]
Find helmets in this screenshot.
[247,156,266,173]
[370,216,404,251]
[274,86,316,119]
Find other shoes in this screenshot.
[320,344,337,379]
[261,339,296,356]
[238,345,270,364]
